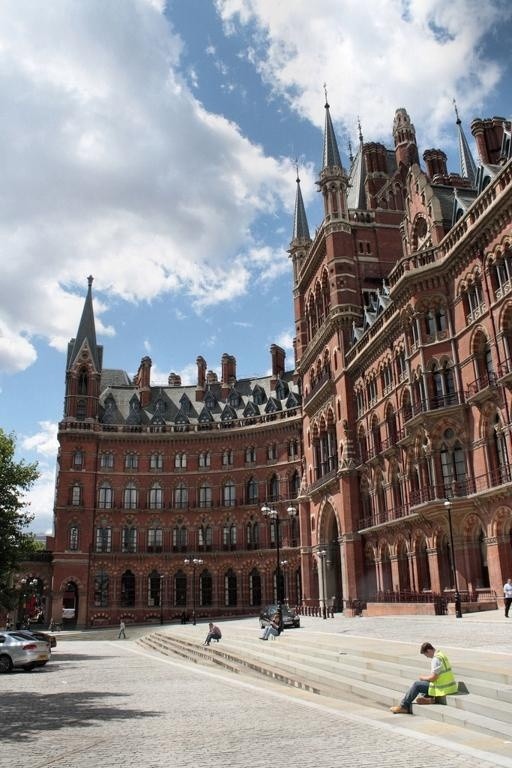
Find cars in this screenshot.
[0,630,53,674]
[259,603,301,629]
[4,629,57,655]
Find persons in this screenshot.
[118,619,126,639]
[5,617,32,630]
[191,609,196,625]
[181,611,186,624]
[391,642,458,714]
[201,623,222,646]
[503,578,512,618]
[259,614,283,640]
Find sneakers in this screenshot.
[203,642,209,646]
[390,706,412,713]
[416,698,435,704]
[259,637,268,641]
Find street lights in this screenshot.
[443,495,461,618]
[22,573,38,630]
[317,549,327,619]
[260,501,298,604]
[185,554,203,626]
[280,560,288,604]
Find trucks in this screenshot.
[37,608,76,620]
